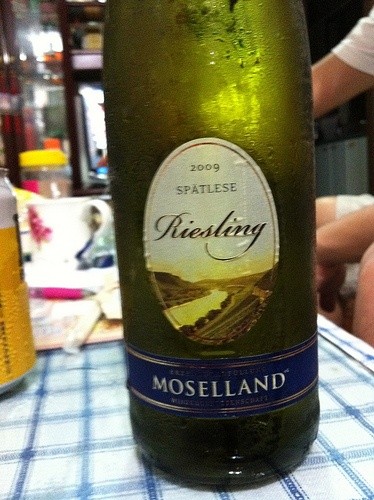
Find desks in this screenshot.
[0,313,374,500]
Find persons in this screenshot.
[307,1,374,350]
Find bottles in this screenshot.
[103,0,319,490]
